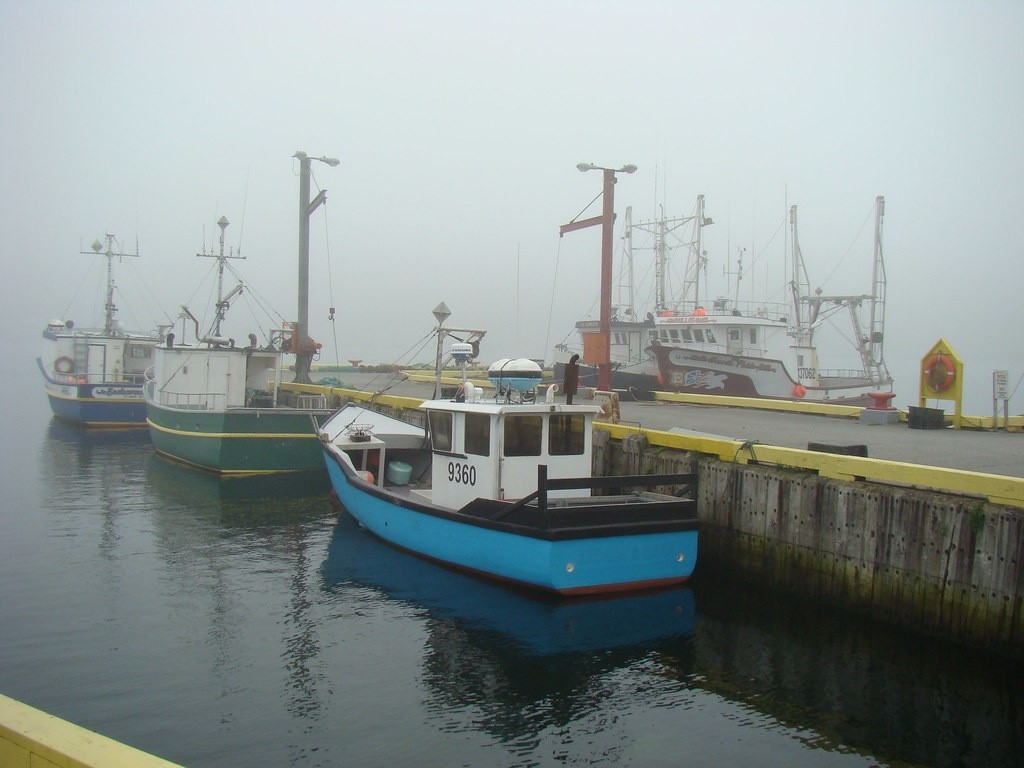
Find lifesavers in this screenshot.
[925,356,955,390]
[54,356,74,374]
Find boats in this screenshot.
[141,216,344,474]
[575,192,898,411]
[44,414,154,551]
[146,457,341,700]
[37,231,156,428]
[318,302,700,594]
[318,520,700,758]
